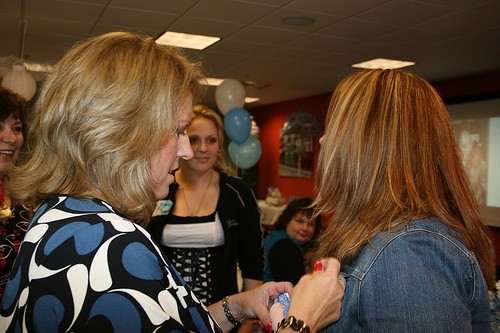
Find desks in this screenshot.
[257,199,286,230]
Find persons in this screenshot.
[0,86,39,308]
[0,31,345,333]
[145,103,263,311]
[263,197,322,288]
[269,69,496,333]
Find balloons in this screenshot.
[215,78,262,170]
[1,70,37,102]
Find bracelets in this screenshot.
[274,316,311,333]
[222,296,244,326]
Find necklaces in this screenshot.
[181,169,213,217]
